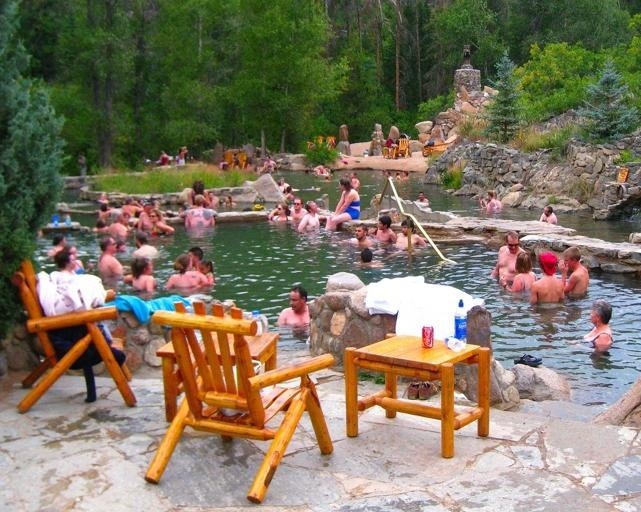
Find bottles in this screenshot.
[455,299,466,344]
[445,336,463,352]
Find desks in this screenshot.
[344,332,491,459]
[155,331,280,423]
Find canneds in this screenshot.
[421,325,435,349]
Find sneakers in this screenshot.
[407,381,437,400]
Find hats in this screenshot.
[540,251,557,274]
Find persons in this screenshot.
[48,143,254,293]
[262,153,430,268]
[582,300,615,353]
[479,190,502,211]
[539,206,557,225]
[489,230,590,305]
[277,285,311,327]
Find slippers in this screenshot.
[514,354,543,365]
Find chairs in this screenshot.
[307,135,336,153]
[383,138,409,159]
[11,271,137,414]
[144,309,336,504]
[20,259,132,387]
